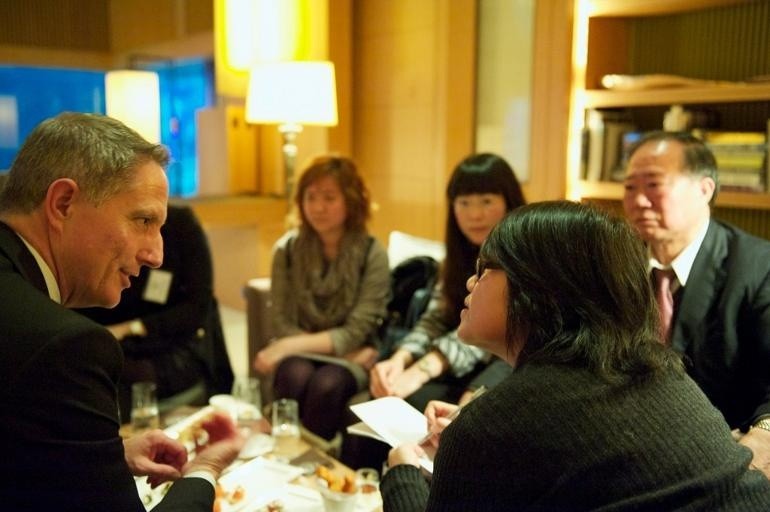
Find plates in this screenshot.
[238,433,274,461]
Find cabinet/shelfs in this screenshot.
[565,1,770,238]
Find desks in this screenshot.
[118,406,382,512]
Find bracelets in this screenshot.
[752,421,770,431]
[416,356,433,380]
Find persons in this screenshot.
[622,131,770,481]
[0,111,244,512]
[339,154,525,472]
[257,155,391,449]
[379,199,770,512]
[75,204,235,406]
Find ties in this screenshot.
[653,268,677,341]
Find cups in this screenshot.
[320,489,360,512]
[357,468,379,485]
[131,384,160,433]
[233,378,262,414]
[272,400,299,446]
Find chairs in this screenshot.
[240,229,447,403]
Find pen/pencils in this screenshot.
[417,384,489,446]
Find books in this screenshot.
[583,108,638,183]
[691,126,766,194]
[345,395,434,478]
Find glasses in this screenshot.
[476,258,504,279]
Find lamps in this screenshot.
[243,61,339,231]
